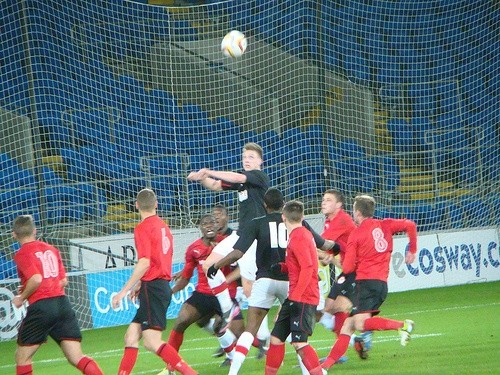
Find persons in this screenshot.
[152,143,375,375]
[111,188,201,375]
[9,214,105,375]
[311,194,417,373]
[263,199,325,375]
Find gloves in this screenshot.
[330,243,340,256]
[207,263,219,279]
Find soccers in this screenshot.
[221,30,247,59]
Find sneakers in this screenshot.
[355,337,369,359]
[319,356,347,363]
[322,368,328,375]
[398,319,414,346]
[361,331,373,352]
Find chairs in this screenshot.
[0,0,500,282]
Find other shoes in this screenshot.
[157,367,176,375]
[258,341,266,359]
[219,358,231,367]
[213,348,224,357]
